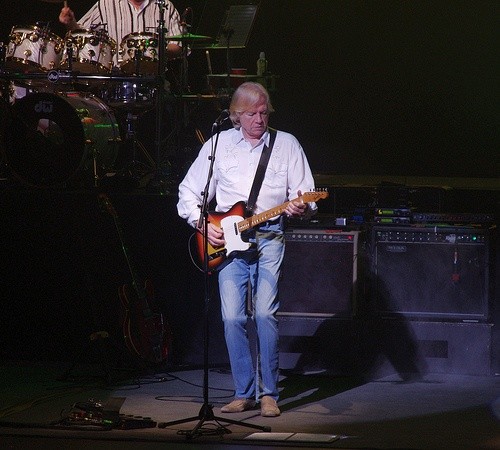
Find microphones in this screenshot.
[214,109,231,127]
[178,8,190,25]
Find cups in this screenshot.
[231,69,247,85]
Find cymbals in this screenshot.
[165,33,213,40]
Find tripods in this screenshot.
[103,52,159,176]
[159,126,271,439]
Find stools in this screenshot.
[174,93,229,184]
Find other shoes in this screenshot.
[261,395,280,417]
[220,399,255,412]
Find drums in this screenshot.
[1,91,120,191]
[103,80,156,110]
[3,23,64,89]
[119,31,160,75]
[59,28,116,84]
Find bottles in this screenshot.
[256,51,268,77]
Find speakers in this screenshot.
[248,228,360,318]
[371,227,491,321]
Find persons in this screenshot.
[176,82,319,418]
[58,0,183,69]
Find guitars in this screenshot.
[97,193,173,362]
[195,187,329,271]
